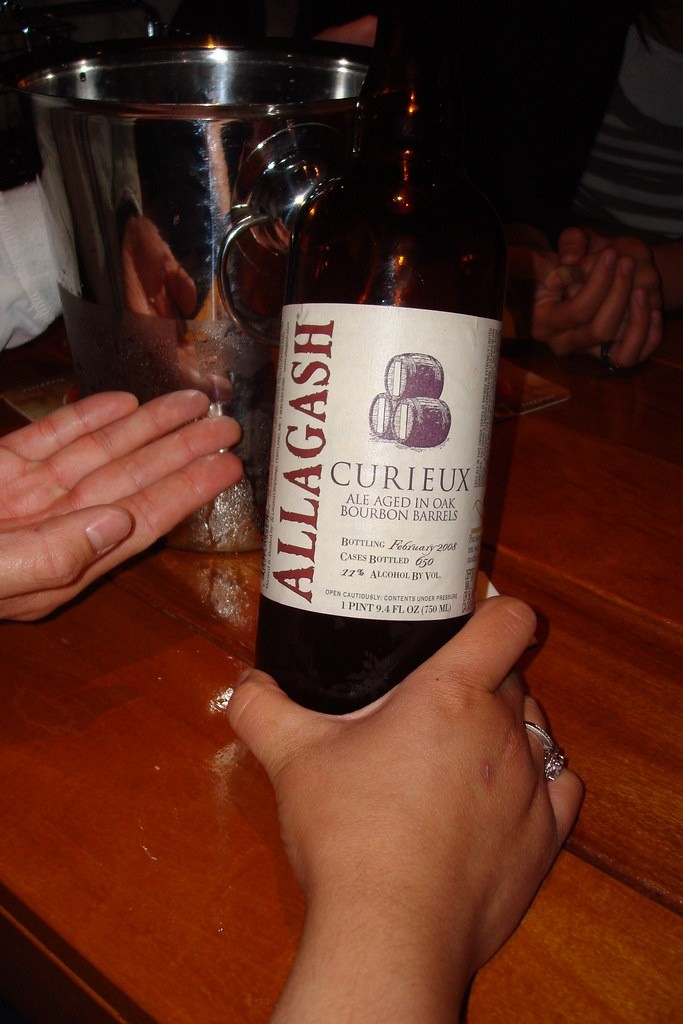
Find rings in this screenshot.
[525,718,564,782]
[601,338,622,374]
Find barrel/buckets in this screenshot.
[19,46,370,554]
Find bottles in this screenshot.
[255,0,513,716]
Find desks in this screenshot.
[0,207,683,1024]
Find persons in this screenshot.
[0,0,683,1024]
